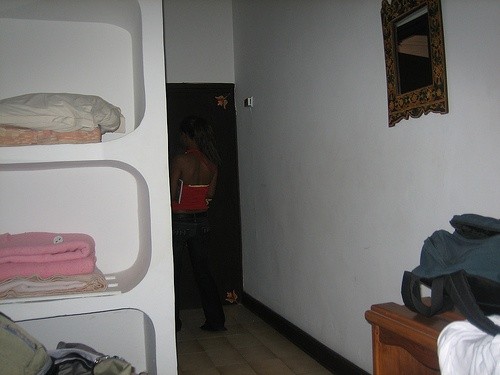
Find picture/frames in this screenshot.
[380,0,449,126]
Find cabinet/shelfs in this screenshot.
[0,0,177,375]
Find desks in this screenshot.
[365,296,464,375]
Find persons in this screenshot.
[169,118,226,330]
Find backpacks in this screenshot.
[399,208,500,333]
